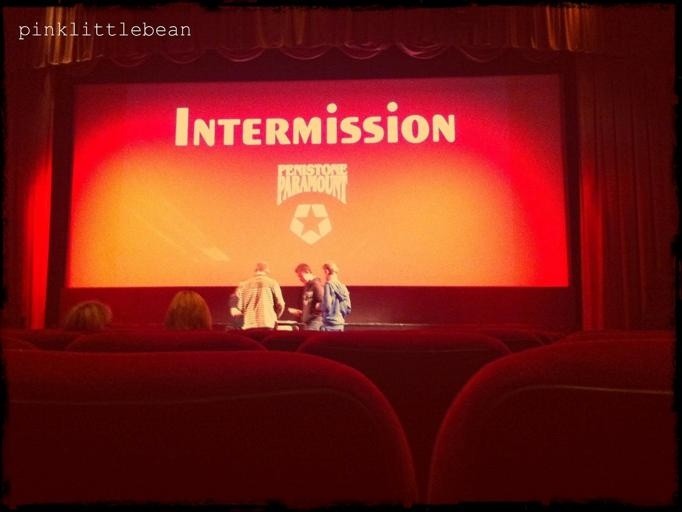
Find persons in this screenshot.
[288,263,324,331]
[315,260,352,332]
[235,262,286,332]
[229,292,242,316]
[64,300,113,331]
[164,290,212,329]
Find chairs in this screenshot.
[1,324,681,512]
